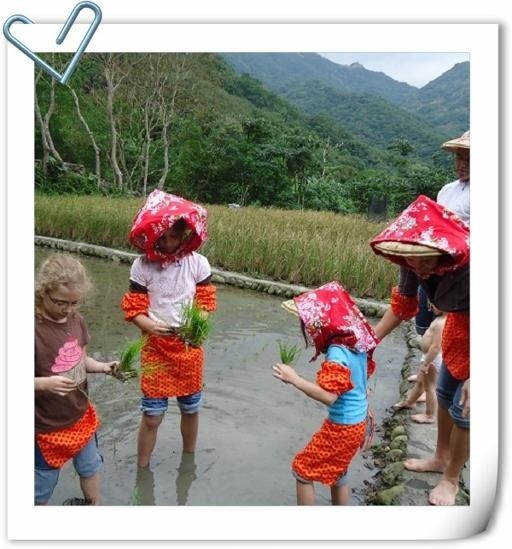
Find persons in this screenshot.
[271,281,381,505]
[373,134,470,505]
[118,187,217,469]
[35,254,139,505]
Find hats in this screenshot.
[441,127,470,152]
[372,240,446,259]
[281,297,299,317]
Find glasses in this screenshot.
[46,291,82,309]
